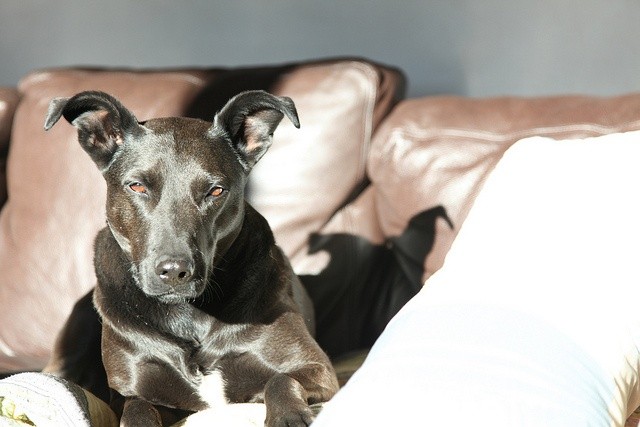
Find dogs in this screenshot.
[41,206,453,427]
[44,90,341,427]
